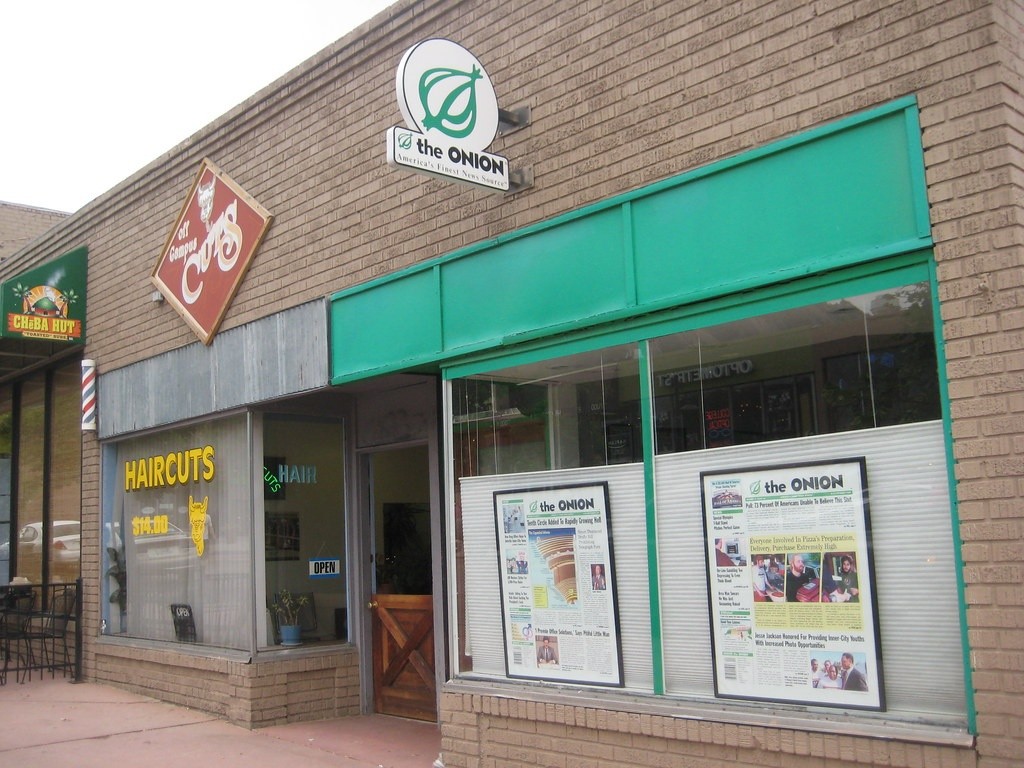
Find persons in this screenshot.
[751,556,784,598]
[811,652,868,691]
[537,636,558,664]
[786,555,809,602]
[822,552,858,602]
[592,565,605,590]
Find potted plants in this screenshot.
[271,589,313,646]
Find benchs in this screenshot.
[274,592,347,643]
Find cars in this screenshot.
[1,519,81,574]
[106,518,200,581]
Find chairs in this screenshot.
[0,589,38,686]
[21,587,77,684]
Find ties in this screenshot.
[546,648,548,661]
[842,670,848,689]
[596,577,599,587]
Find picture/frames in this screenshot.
[699,456,887,712]
[493,481,625,689]
